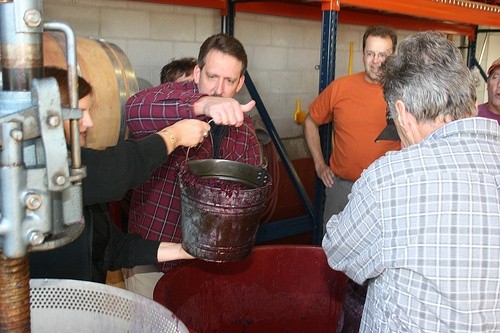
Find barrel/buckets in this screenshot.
[42,32,140,150]
[178,119,273,263]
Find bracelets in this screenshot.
[159,129,177,153]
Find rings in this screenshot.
[203,130,209,137]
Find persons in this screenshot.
[28,65,211,284]
[322,31,500,333]
[160,57,197,84]
[477,58,500,125]
[121,33,262,301]
[304,25,401,236]
